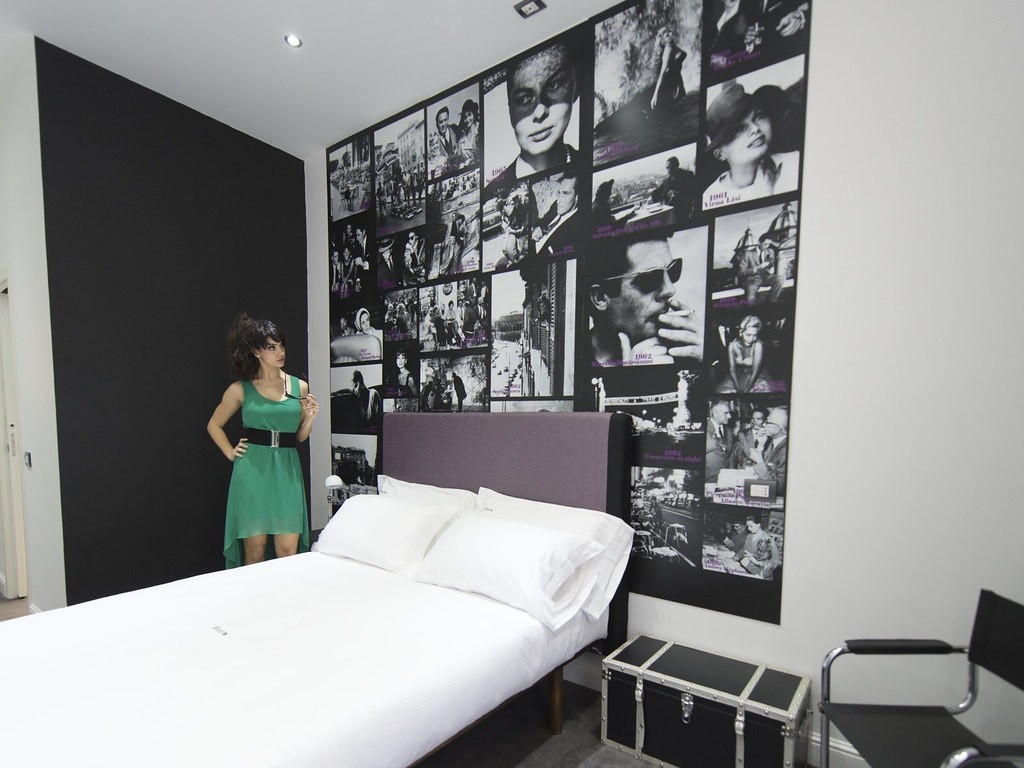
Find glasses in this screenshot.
[408,235,416,240]
[765,420,779,426]
[285,373,309,400]
[604,258,682,293]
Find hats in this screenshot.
[379,238,394,252]
[495,199,506,211]
[705,84,789,152]
[459,100,478,128]
[356,308,370,329]
[511,194,521,204]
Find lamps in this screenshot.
[324,476,343,523]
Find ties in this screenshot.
[771,440,775,449]
[388,255,392,269]
[754,433,759,449]
[719,427,724,441]
[545,216,561,234]
[759,252,762,264]
[443,135,450,152]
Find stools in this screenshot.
[630,505,688,563]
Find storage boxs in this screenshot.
[601,633,812,768]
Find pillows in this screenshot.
[310,475,478,579]
[415,487,635,632]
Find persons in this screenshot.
[329,0,812,582]
[207,313,320,570]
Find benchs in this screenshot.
[421,333,435,349]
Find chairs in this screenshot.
[339,193,352,211]
[460,321,482,347]
[819,587,1024,768]
[360,173,372,210]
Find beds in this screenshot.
[0,412,636,768]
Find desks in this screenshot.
[625,203,674,234]
